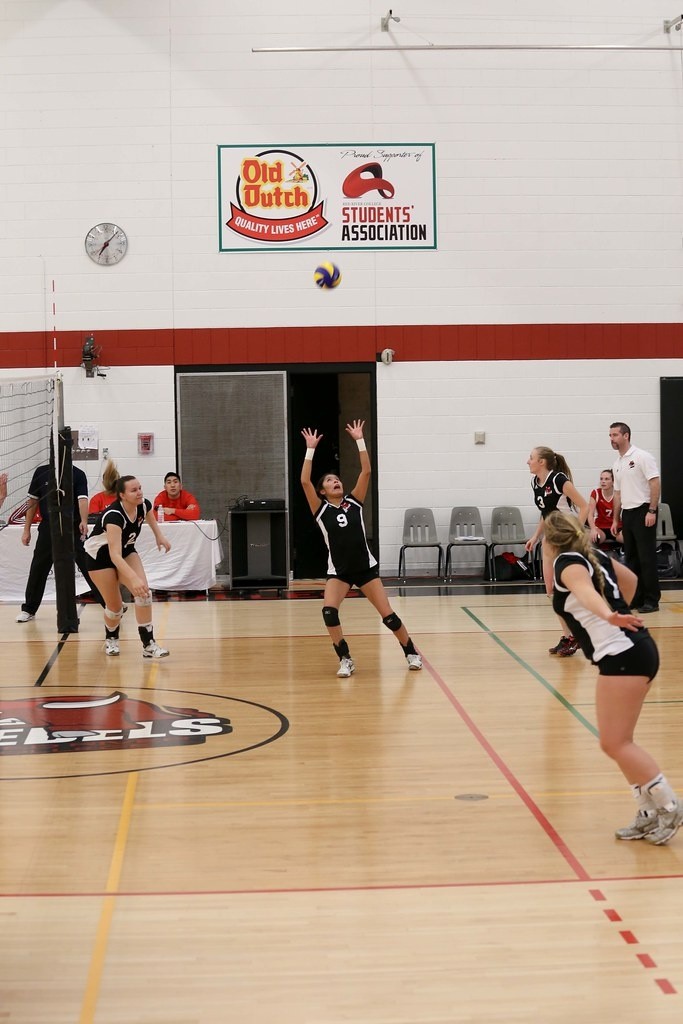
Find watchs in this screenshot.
[648,509,657,514]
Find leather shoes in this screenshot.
[637,603,659,613]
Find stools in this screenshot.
[599,538,616,544]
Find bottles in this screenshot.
[157,504,164,524]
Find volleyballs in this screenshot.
[313,260,342,289]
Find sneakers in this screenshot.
[556,636,580,657]
[142,639,169,658]
[616,806,660,839]
[120,601,129,612]
[105,631,120,656]
[16,611,33,621]
[549,636,570,654]
[644,800,683,845]
[406,654,423,670]
[336,658,355,677]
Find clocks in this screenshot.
[85,223,128,266]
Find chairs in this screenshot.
[443,506,493,582]
[397,507,445,581]
[489,506,537,581]
[653,504,681,578]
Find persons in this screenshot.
[153,471,200,595]
[80,462,171,658]
[588,469,624,545]
[8,499,42,525]
[609,422,661,613]
[15,464,89,623]
[301,419,422,678]
[525,446,591,656]
[542,512,683,845]
[89,473,117,513]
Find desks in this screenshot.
[0,519,223,596]
